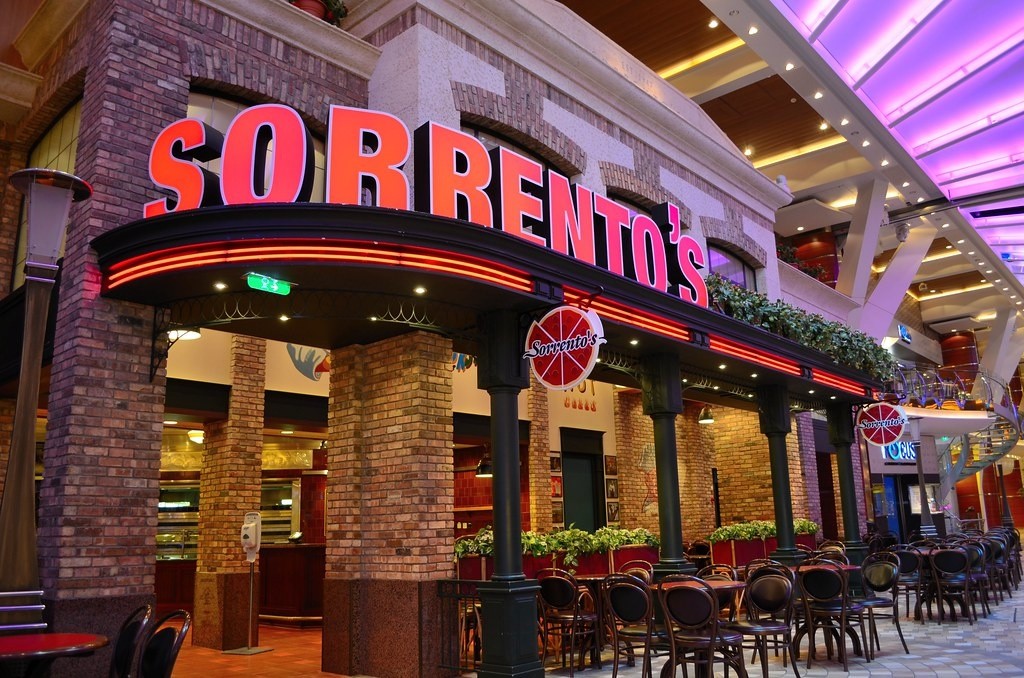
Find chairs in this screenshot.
[460,526,1024,678]
[108,603,152,678]
[136,608,191,678]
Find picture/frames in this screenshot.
[607,502,620,522]
[606,478,619,498]
[605,455,618,475]
[550,451,561,472]
[551,476,563,497]
[552,501,563,523]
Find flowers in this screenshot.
[288,0,349,28]
[776,242,826,280]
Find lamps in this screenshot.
[188,430,204,445]
[474,449,493,478]
[168,325,201,340]
[697,404,715,424]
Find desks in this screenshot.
[564,573,635,672]
[648,581,748,678]
[779,566,863,661]
[912,549,969,621]
[0,632,108,678]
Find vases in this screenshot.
[292,0,327,19]
